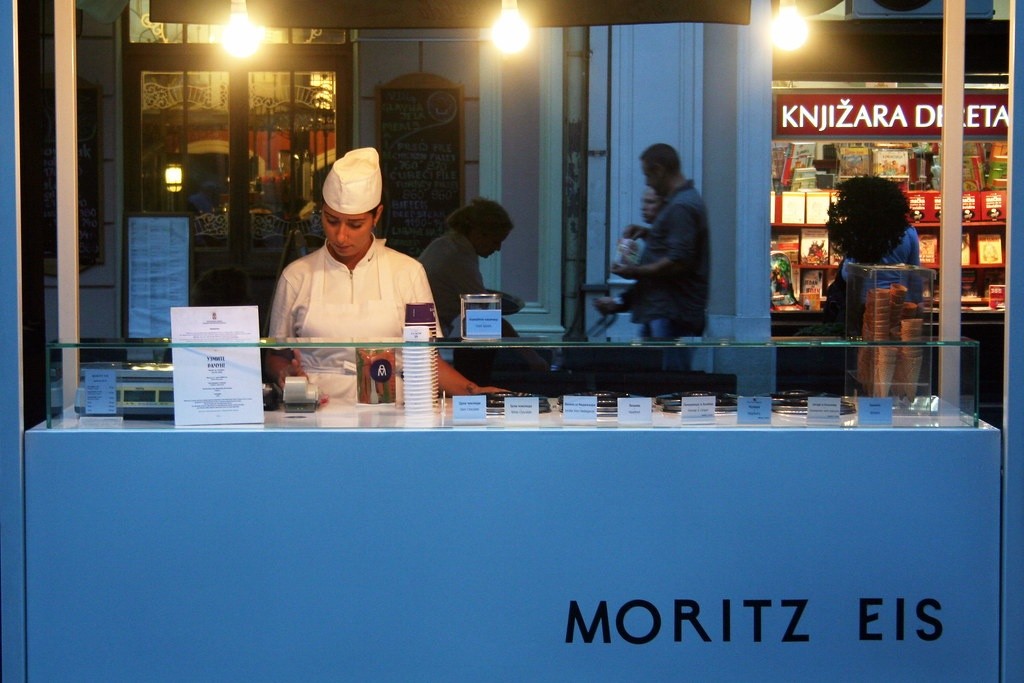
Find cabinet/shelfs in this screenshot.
[771,137,1005,328]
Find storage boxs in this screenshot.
[844,262,935,416]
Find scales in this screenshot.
[115,361,176,416]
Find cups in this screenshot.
[460,293,503,341]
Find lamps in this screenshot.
[163,152,184,193]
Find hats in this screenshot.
[321,146,383,215]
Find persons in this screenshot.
[263,144,511,404]
[610,143,709,368]
[590,190,673,340]
[410,195,549,372]
[180,175,287,246]
[838,223,924,395]
